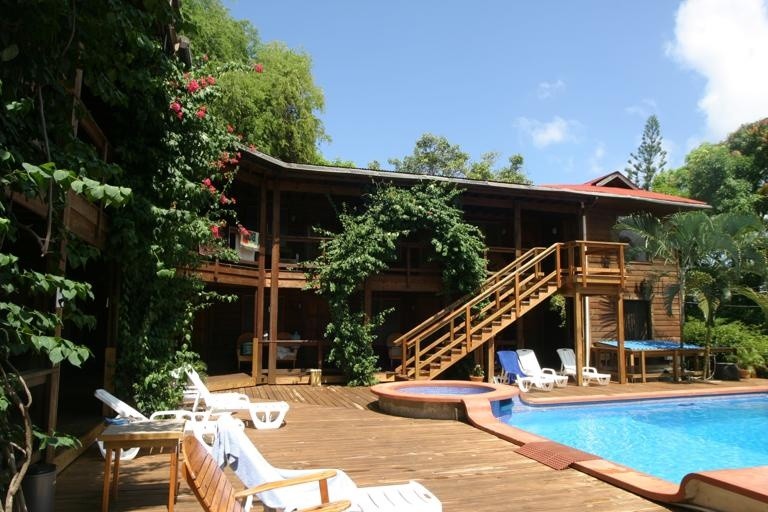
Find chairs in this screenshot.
[494,343,613,394]
[230,327,304,372]
[386,333,409,368]
[215,414,442,512]
[182,418,444,512]
[87,365,290,460]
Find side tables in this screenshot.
[95,423,186,512]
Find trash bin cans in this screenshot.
[19,463,56,512]
